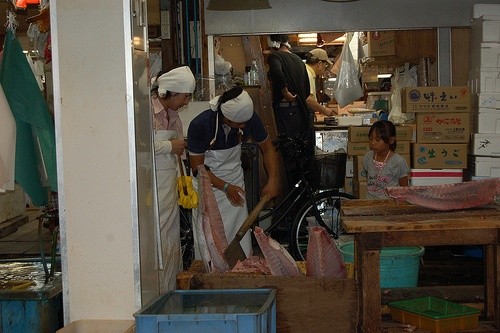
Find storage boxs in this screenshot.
[414,112,472,144]
[55,318,135,333]
[344,122,412,140]
[132,288,277,333]
[471,3,500,181]
[409,166,464,186]
[387,294,482,333]
[351,155,412,180]
[345,175,353,193]
[410,141,469,169]
[358,180,367,199]
[346,139,410,156]
[365,30,397,58]
[344,154,354,178]
[401,83,471,114]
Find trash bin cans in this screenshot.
[338,241,425,286]
[132,287,277,333]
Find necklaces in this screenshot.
[373,149,391,191]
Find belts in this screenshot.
[278,101,299,108]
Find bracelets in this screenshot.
[223,183,229,192]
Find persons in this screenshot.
[267,33,322,189]
[304,47,334,161]
[151,63,196,293]
[187,86,280,272]
[358,119,410,199]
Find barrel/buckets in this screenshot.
[337,241,425,287]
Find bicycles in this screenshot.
[180,127,359,263]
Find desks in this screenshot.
[337,197,500,318]
[313,122,350,154]
[338,211,500,333]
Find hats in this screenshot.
[307,48,334,66]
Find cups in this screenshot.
[244,66,250,85]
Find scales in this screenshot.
[369,92,392,115]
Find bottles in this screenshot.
[250,60,259,86]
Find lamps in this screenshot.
[296,32,317,44]
[375,73,392,79]
[317,32,324,48]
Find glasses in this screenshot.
[321,61,329,68]
